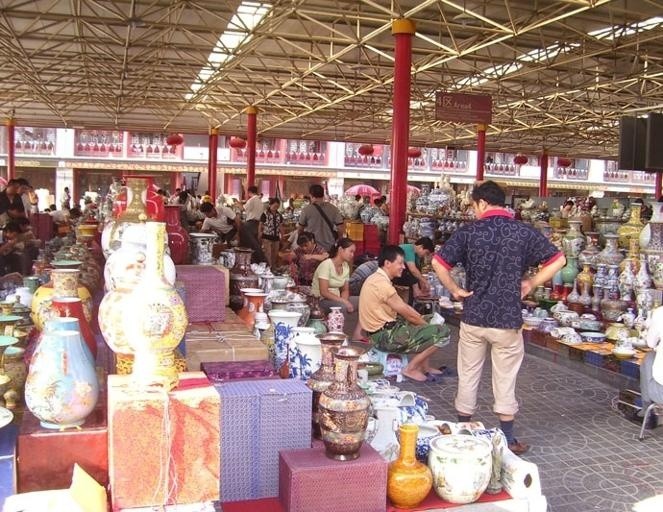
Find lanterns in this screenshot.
[560,157,571,170]
[228,136,247,154]
[405,147,425,160]
[515,153,530,166]
[165,134,185,151]
[356,144,374,159]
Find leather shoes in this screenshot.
[622,406,656,429]
[508,437,530,454]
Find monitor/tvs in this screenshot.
[645,111,663,171]
[617,114,647,170]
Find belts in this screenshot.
[365,327,386,335]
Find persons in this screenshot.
[626,304,663,429]
[0,176,82,272]
[358,245,451,383]
[169,185,285,274]
[287,184,434,345]
[636,199,651,218]
[562,200,573,211]
[431,181,567,454]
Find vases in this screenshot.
[332,173,663,331]
[390,423,432,509]
[0,175,216,434]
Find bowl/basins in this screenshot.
[612,349,636,360]
[580,332,606,344]
[523,316,544,326]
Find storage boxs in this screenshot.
[1,412,113,511]
[107,263,388,511]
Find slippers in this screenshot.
[410,372,444,383]
[429,366,457,377]
